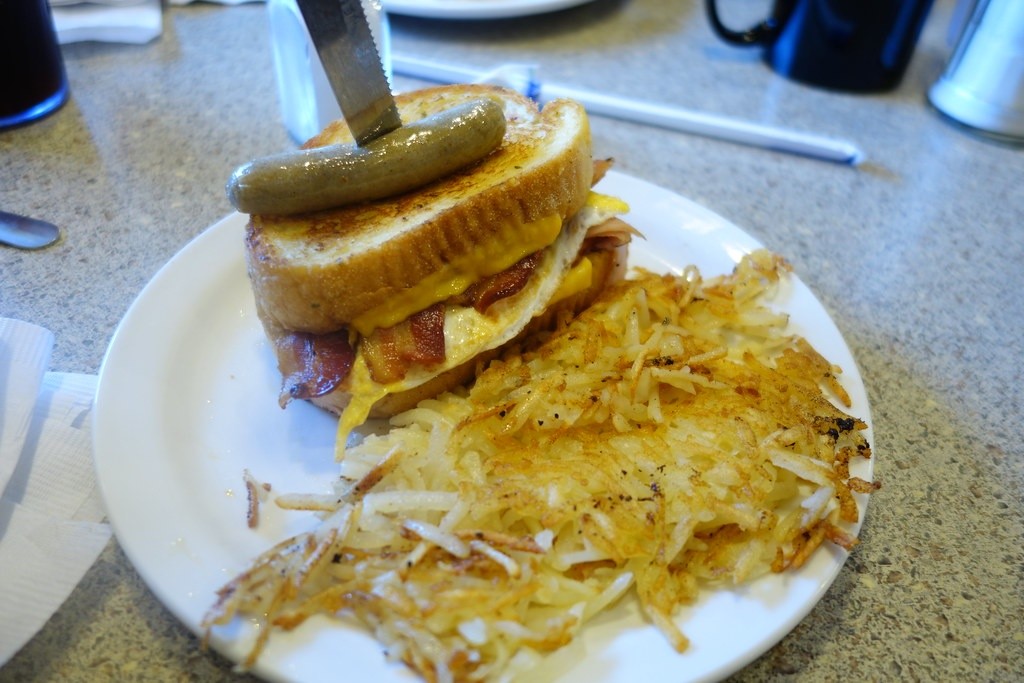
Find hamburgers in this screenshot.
[244,82,644,460]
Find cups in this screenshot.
[702,1,935,95]
[0,0,70,130]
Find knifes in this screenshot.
[296,2,401,147]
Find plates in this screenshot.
[88,168,877,683]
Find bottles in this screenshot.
[924,0,1024,147]
[264,1,402,148]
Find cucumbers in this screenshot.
[224,97,508,214]
